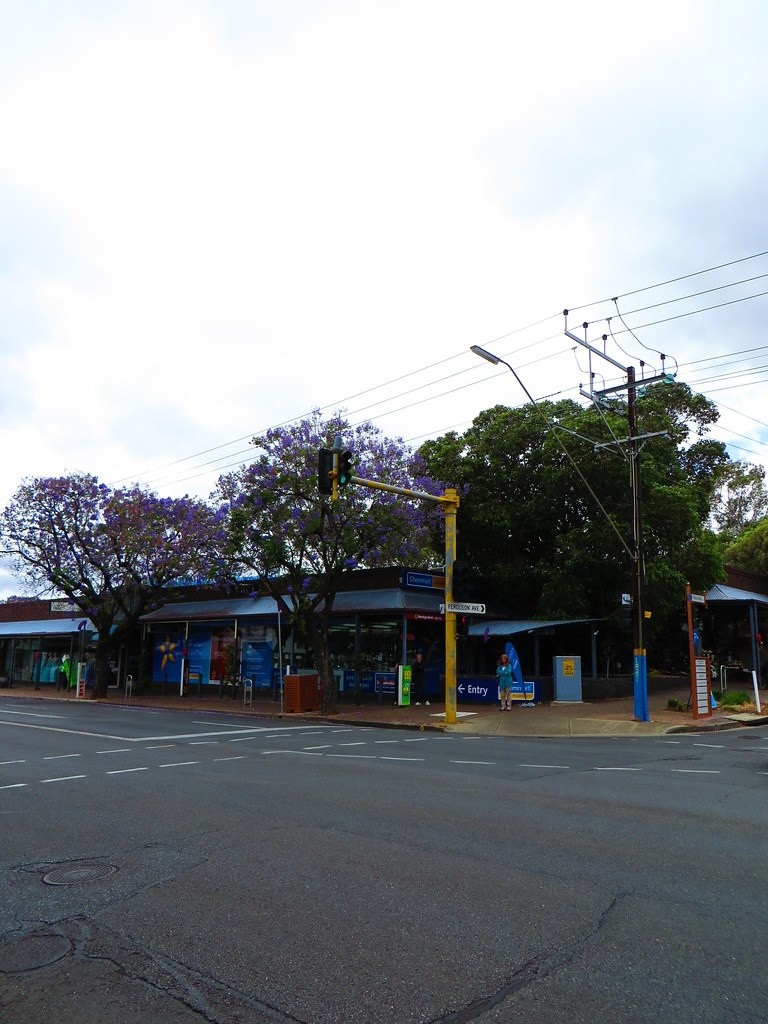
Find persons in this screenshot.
[412,651,430,705]
[667,648,734,680]
[331,641,385,670]
[393,654,403,705]
[33,651,60,682]
[495,654,511,711]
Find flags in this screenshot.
[505,642,522,685]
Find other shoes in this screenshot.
[500,706,506,711]
[425,701,431,706]
[415,702,421,706]
[507,707,512,711]
